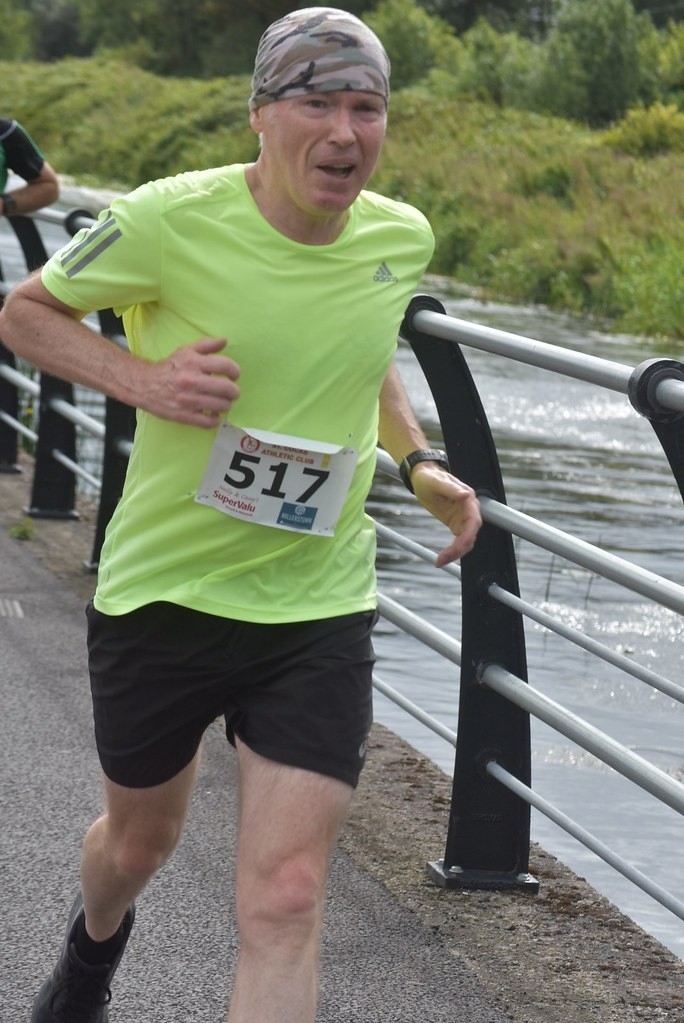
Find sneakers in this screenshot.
[29,890,138,1023]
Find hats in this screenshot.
[249,7,392,114]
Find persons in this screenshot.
[0,7,482,1023]
[0,118,60,215]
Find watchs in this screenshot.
[400,448,450,496]
